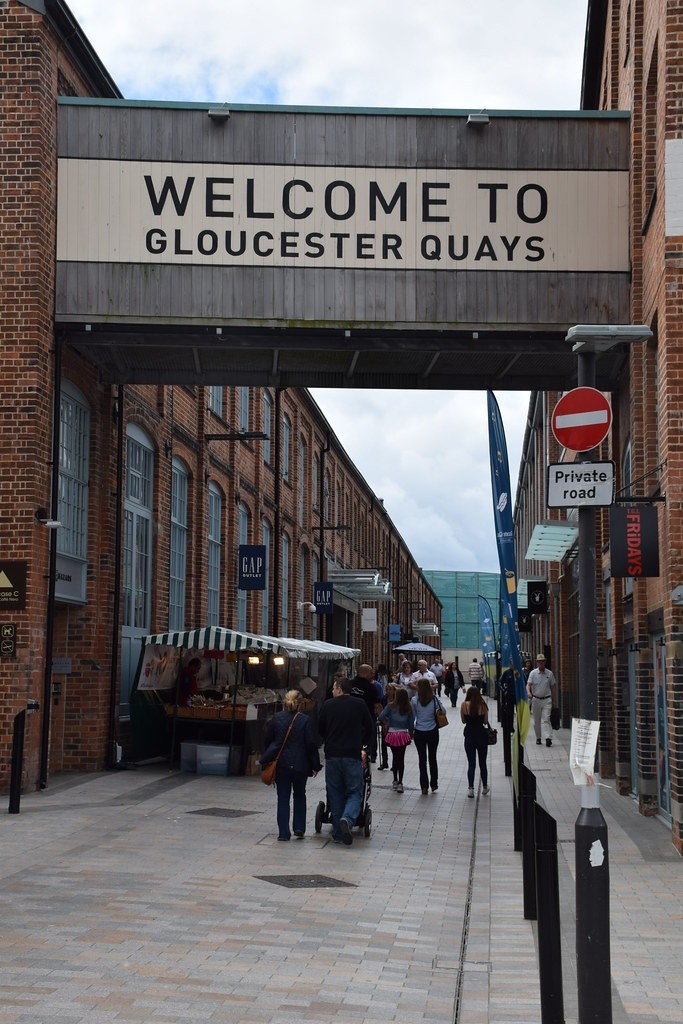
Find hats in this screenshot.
[534,654,546,660]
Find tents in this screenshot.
[143,625,362,744]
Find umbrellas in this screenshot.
[392,639,442,663]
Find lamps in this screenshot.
[205,431,270,440]
[35,508,63,529]
[208,102,231,121]
[465,109,489,127]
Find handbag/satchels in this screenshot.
[261,760,277,786]
[483,722,497,745]
[434,695,449,729]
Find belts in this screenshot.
[533,695,551,700]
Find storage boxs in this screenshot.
[179,738,244,777]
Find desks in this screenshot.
[163,706,317,777]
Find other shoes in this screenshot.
[277,837,290,841]
[333,834,342,842]
[339,818,353,845]
[377,763,389,770]
[371,755,376,763]
[482,788,490,794]
[392,780,399,790]
[396,784,403,793]
[422,789,428,794]
[468,790,474,798]
[431,785,438,792]
[546,739,552,747]
[536,738,541,744]
[293,831,305,839]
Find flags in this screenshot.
[476,388,531,745]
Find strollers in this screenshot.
[314,743,374,836]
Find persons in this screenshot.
[176,653,559,844]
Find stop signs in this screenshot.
[552,387,613,453]
